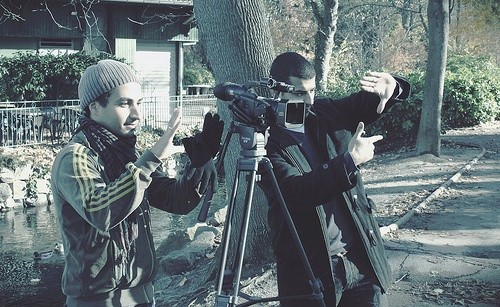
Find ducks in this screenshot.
[34,242,65,261]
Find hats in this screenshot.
[78,58,140,111]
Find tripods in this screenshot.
[207,131,327,307]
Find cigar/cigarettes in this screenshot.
[307,109,316,117]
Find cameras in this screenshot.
[212,77,307,131]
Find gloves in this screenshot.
[181,112,225,190]
[181,132,219,192]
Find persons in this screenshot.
[253,52,412,307]
[51,59,225,306]
